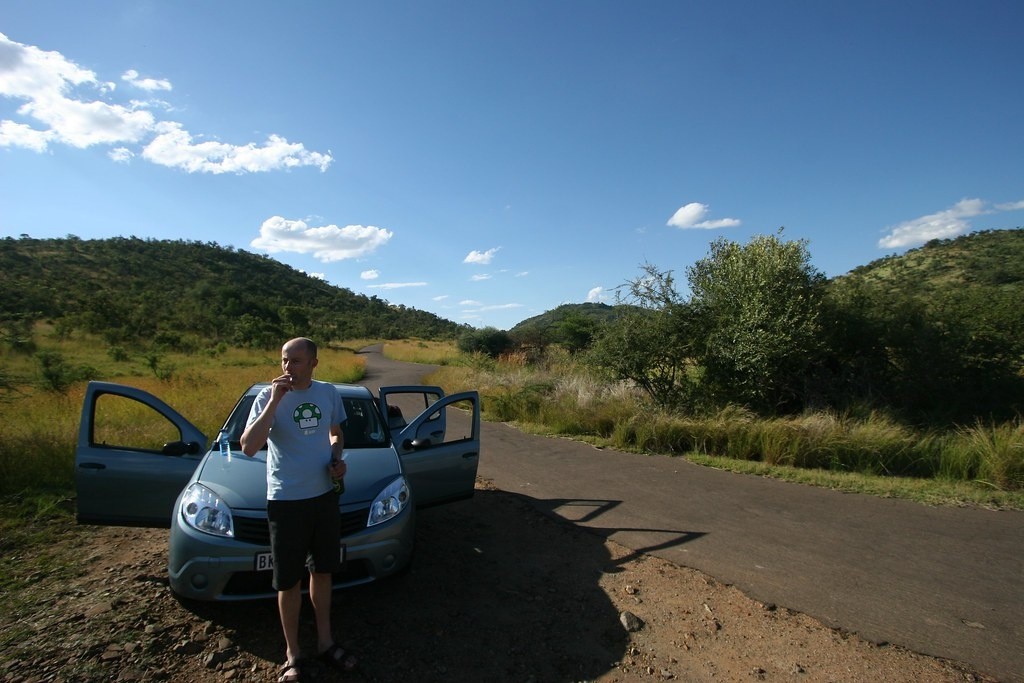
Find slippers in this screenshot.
[280,651,302,683]
[314,640,360,673]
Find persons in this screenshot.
[240,336,359,683]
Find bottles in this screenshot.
[331,458,344,495]
[220,429,231,462]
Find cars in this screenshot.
[75,378,482,629]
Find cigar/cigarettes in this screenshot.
[290,377,293,381]
